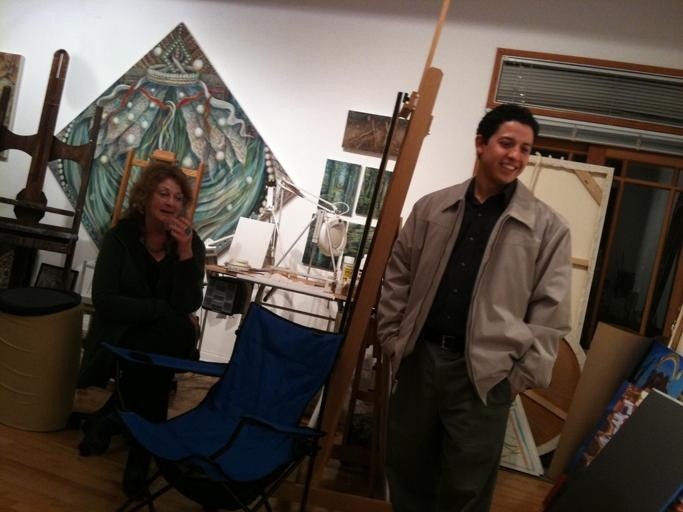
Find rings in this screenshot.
[182,225,189,235]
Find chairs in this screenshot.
[97,300,347,512]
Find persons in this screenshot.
[75,159,205,501]
[375,103,574,511]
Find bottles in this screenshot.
[324,276,337,294]
[342,255,355,289]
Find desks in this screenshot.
[197,262,350,349]
